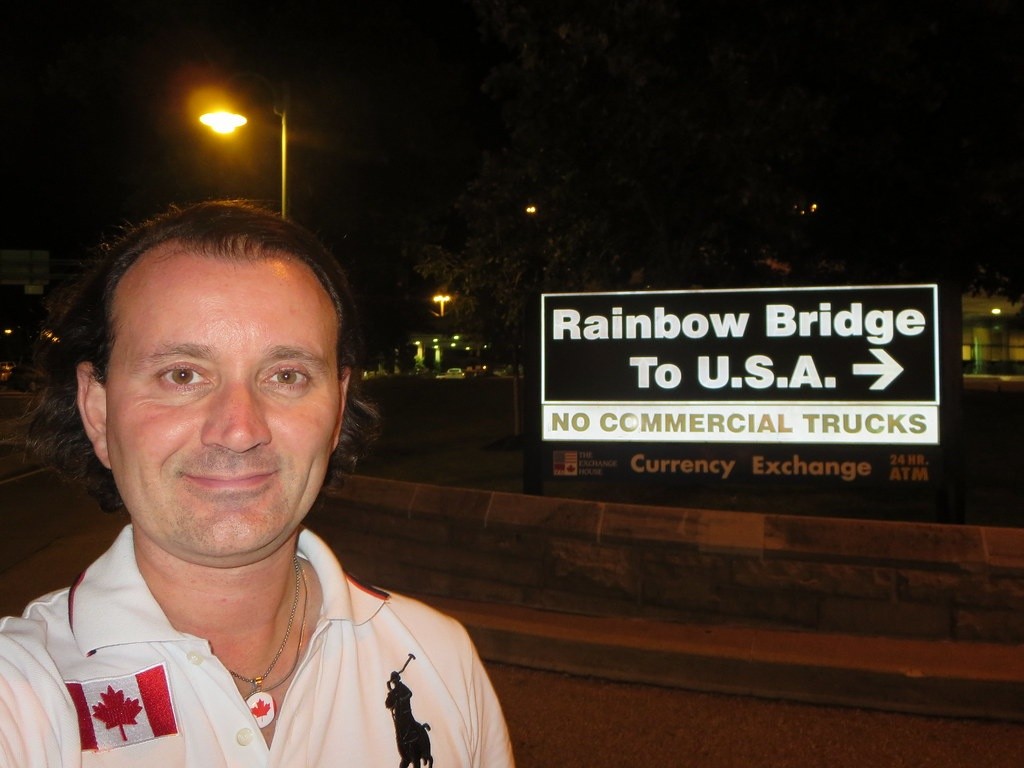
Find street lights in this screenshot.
[198,92,290,219]
[513,342,521,437]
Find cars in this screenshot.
[0,361,16,371]
[436,368,477,380]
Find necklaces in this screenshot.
[227,555,307,729]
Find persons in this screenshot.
[0,198,519,768]
